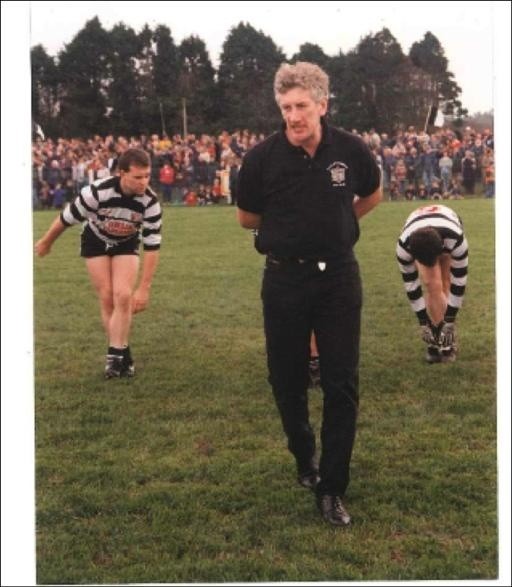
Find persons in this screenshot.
[394,201,472,364]
[34,127,266,209]
[33,146,164,381]
[233,60,387,527]
[353,125,495,201]
[307,328,322,388]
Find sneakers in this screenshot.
[125,347,135,377]
[104,353,125,379]
[442,348,455,363]
[425,348,440,362]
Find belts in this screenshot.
[258,252,354,273]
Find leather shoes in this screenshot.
[296,457,321,490]
[314,490,350,527]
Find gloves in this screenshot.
[439,321,456,346]
[420,321,438,346]
